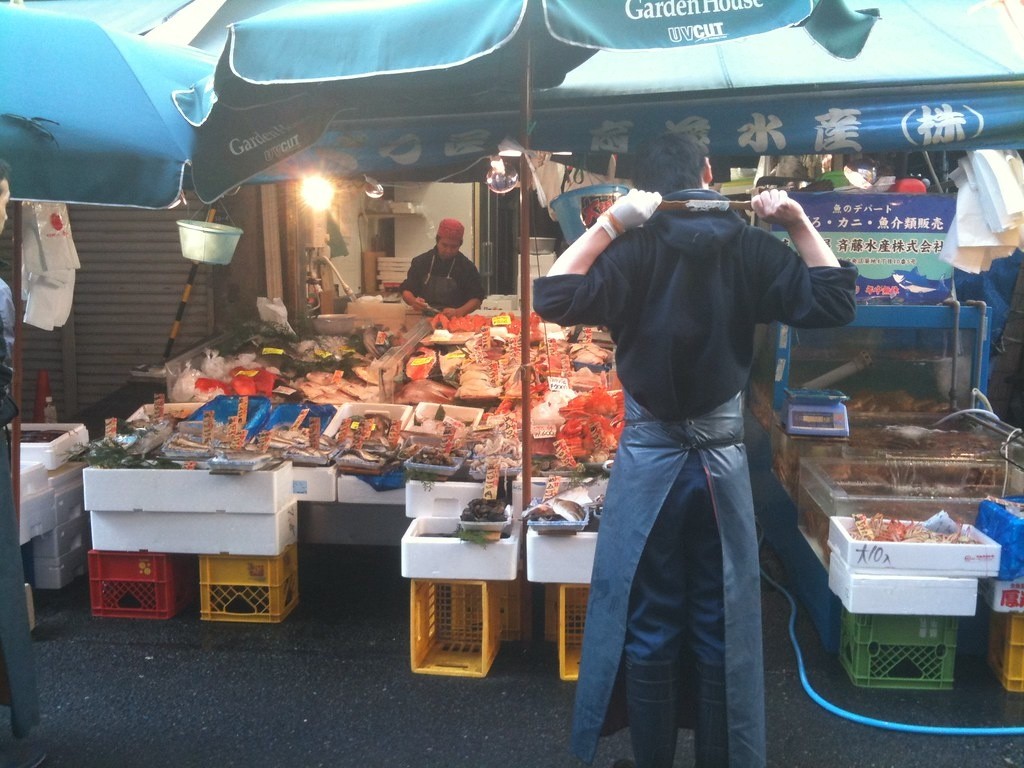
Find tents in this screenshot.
[244,0,1024,186]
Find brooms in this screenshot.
[61,199,221,442]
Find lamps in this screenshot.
[364,178,384,197]
[485,156,519,194]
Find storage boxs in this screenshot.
[2,395,600,682]
[745,304,1024,693]
[482,253,557,311]
[346,250,422,324]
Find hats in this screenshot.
[437,218,464,240]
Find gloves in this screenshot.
[595,189,663,240]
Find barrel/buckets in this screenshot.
[176,220,244,265]
[549,183,630,245]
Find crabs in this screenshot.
[557,388,624,463]
[431,313,542,339]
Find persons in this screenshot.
[398,219,486,319]
[531,128,858,768]
[0,159,40,742]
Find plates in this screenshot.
[720,178,753,201]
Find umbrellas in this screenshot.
[171,0,885,768]
[0,0,241,210]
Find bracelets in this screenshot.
[603,209,624,235]
[596,216,617,241]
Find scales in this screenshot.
[780,387,850,437]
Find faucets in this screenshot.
[341,282,353,296]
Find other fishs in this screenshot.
[883,426,958,445]
[518,477,598,521]
[339,442,395,462]
[167,429,335,460]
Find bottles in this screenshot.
[43,396,60,423]
